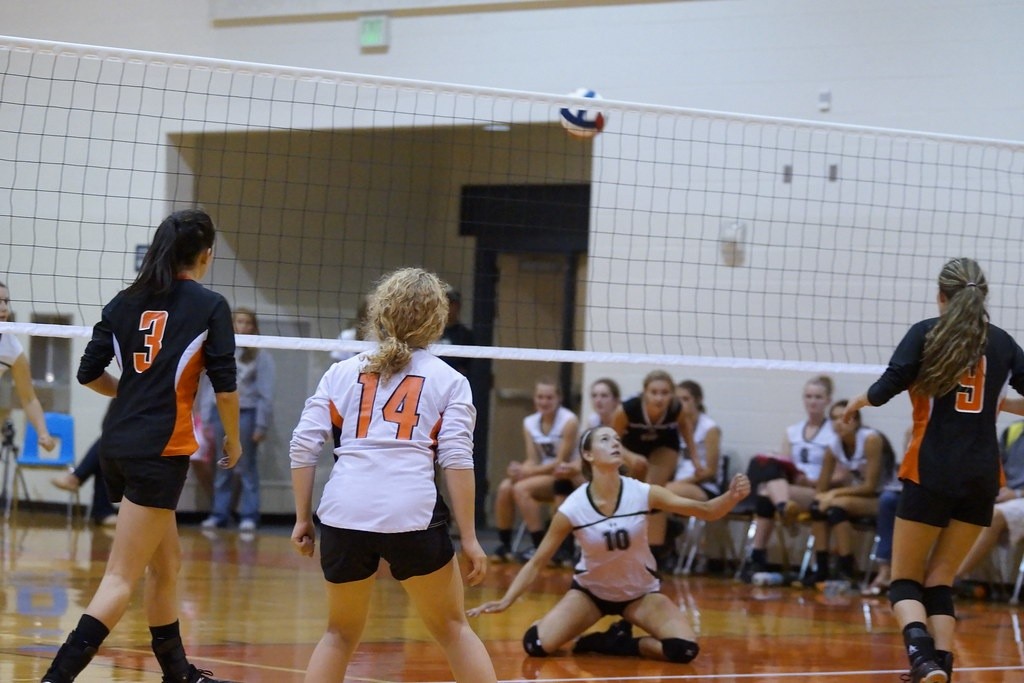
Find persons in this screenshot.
[741,376,913,597]
[0,283,55,452]
[42,210,243,683]
[465,426,750,664]
[843,258,1024,683]
[954,418,1024,582]
[50,436,117,525]
[200,309,275,531]
[290,269,499,683]
[493,370,721,581]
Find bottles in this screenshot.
[817,581,849,593]
[753,571,785,586]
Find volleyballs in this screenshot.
[559,87,610,138]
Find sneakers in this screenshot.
[900,659,948,683]
[572,618,634,655]
[160,663,239,683]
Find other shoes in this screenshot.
[50,475,80,493]
[833,571,859,591]
[505,545,537,563]
[237,518,259,530]
[488,550,513,563]
[777,501,801,539]
[663,554,678,574]
[950,575,971,599]
[202,517,228,529]
[739,560,769,582]
[100,513,118,526]
[861,578,891,595]
[546,554,573,566]
[793,570,832,589]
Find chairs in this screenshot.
[3,412,81,527]
[671,453,882,594]
